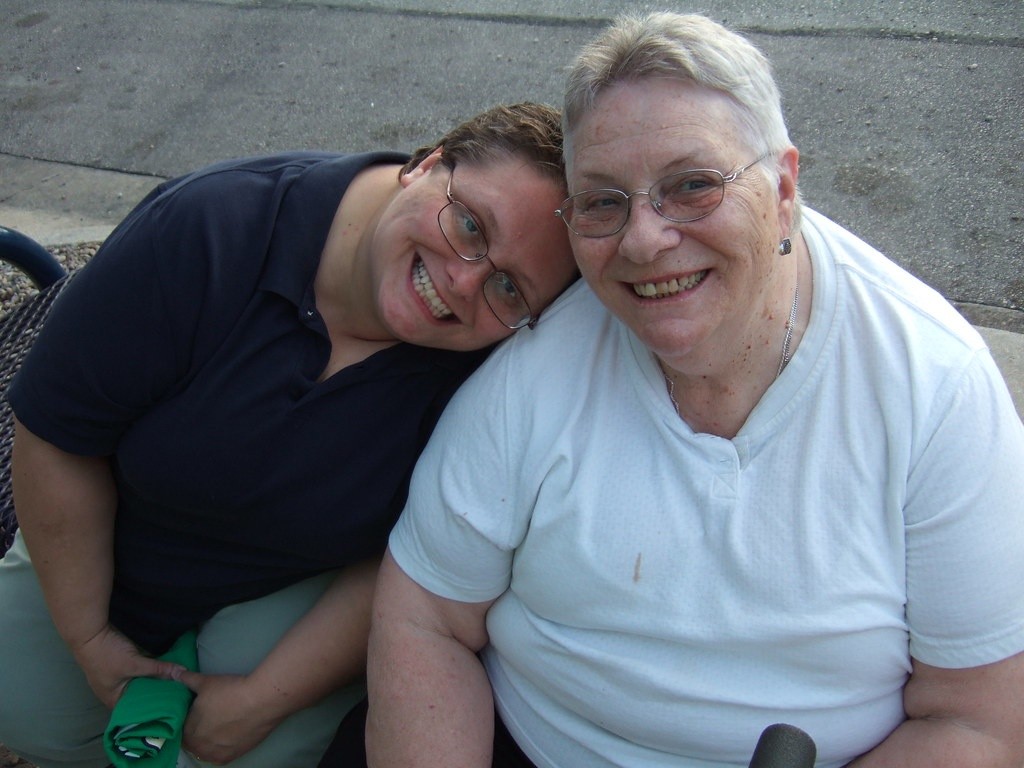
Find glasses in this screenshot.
[438,166,540,330]
[553,149,777,239]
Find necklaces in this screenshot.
[662,281,799,417]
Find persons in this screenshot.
[0,103,584,768]
[364,9,1023,768]
